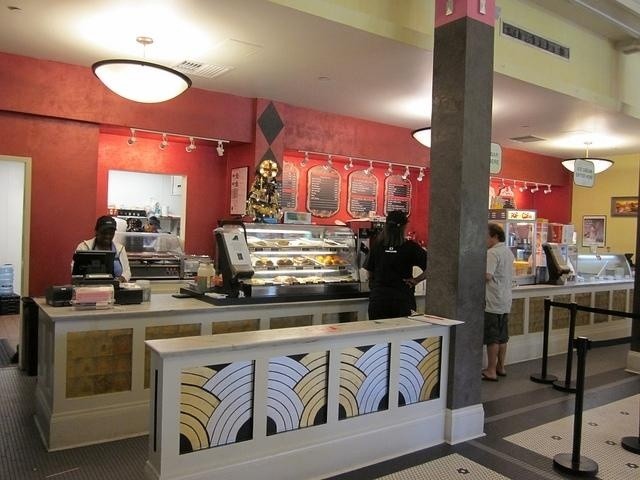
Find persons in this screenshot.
[128,216,161,251]
[70,215,131,284]
[479,222,516,381]
[362,210,426,320]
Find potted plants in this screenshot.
[582,215,606,247]
[611,196,640,217]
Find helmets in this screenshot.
[302,254,353,266]
[299,237,341,247]
[252,253,325,267]
[263,238,319,248]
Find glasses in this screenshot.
[91,60,192,104]
[561,158,616,174]
[413,127,439,149]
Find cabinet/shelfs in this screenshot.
[208,264,215,289]
[517,237,533,262]
[198,264,207,292]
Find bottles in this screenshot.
[95,216,116,231]
[146,216,161,229]
[386,211,410,226]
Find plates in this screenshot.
[31,277,636,453]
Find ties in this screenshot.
[70,249,115,284]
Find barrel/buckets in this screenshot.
[498,373,506,376]
[481,373,498,382]
